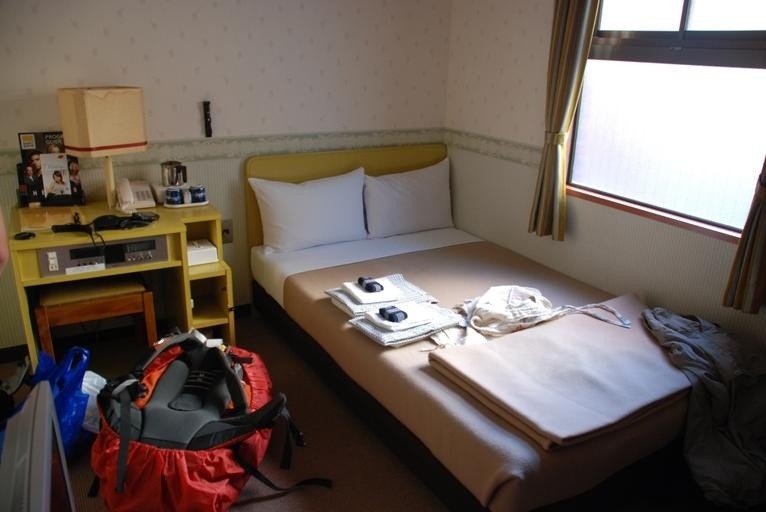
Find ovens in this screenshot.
[244,142,733,512]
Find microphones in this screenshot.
[34,282,160,363]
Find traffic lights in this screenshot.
[248,156,455,259]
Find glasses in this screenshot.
[59,86,148,210]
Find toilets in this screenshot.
[115,177,156,210]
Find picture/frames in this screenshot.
[7,202,237,374]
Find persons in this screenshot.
[29,151,44,190]
[46,171,70,199]
[24,166,35,186]
[69,159,81,191]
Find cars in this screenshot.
[167,185,205,205]
[160,160,187,187]
